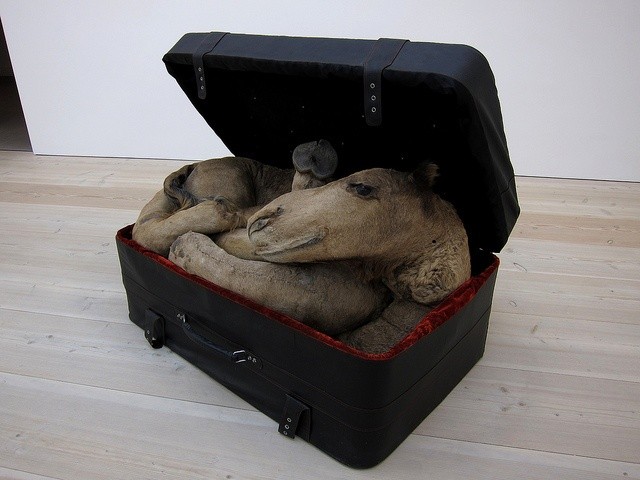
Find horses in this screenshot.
[132,137,472,355]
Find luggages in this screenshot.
[116,32,520,469]
